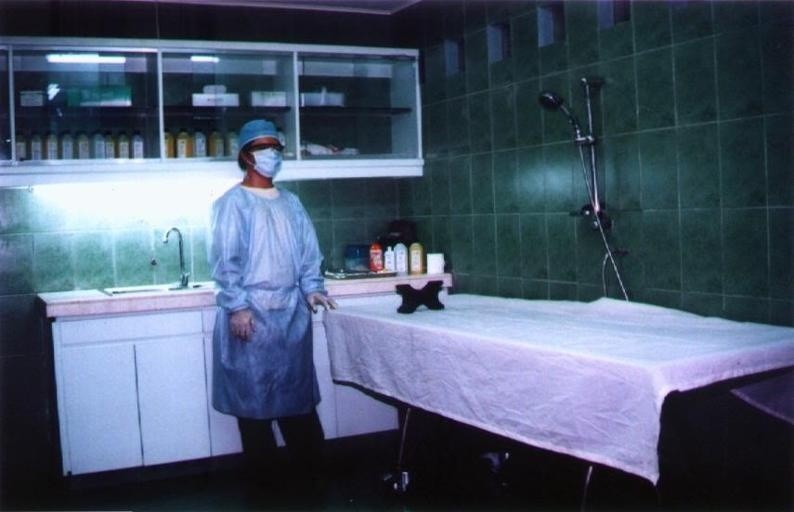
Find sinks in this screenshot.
[102,282,213,297]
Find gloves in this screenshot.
[308,294,337,313]
[230,312,256,342]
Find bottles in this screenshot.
[16,128,241,161]
[408,239,424,275]
[394,240,408,275]
[384,246,395,273]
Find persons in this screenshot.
[205,116,341,512]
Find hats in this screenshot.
[238,119,279,171]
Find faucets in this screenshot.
[161,227,190,289]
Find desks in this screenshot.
[317,292,792,512]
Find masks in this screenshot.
[243,149,283,178]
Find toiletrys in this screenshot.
[384,246,394,270]
[409,242,423,273]
[394,243,409,276]
[427,253,446,274]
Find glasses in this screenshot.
[250,143,283,151]
